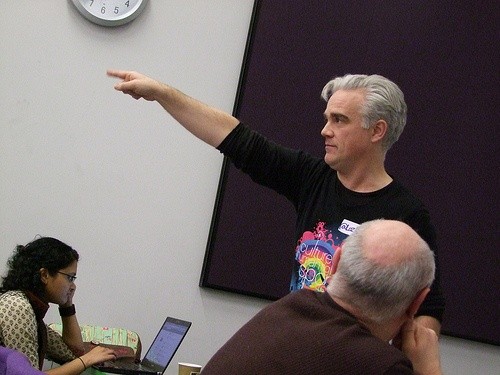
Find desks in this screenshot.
[40,342,135,375]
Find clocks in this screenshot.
[71,0,147,26]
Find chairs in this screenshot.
[49,323,142,361]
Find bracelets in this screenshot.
[58,304,76,316]
[78,357,86,371]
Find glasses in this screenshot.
[39,267,77,282]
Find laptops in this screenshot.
[91,317,192,375]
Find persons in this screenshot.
[106,69,446,344]
[0,237,116,375]
[199,219,442,375]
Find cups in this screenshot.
[178,362,202,375]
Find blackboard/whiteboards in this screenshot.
[198,0,499,348]
